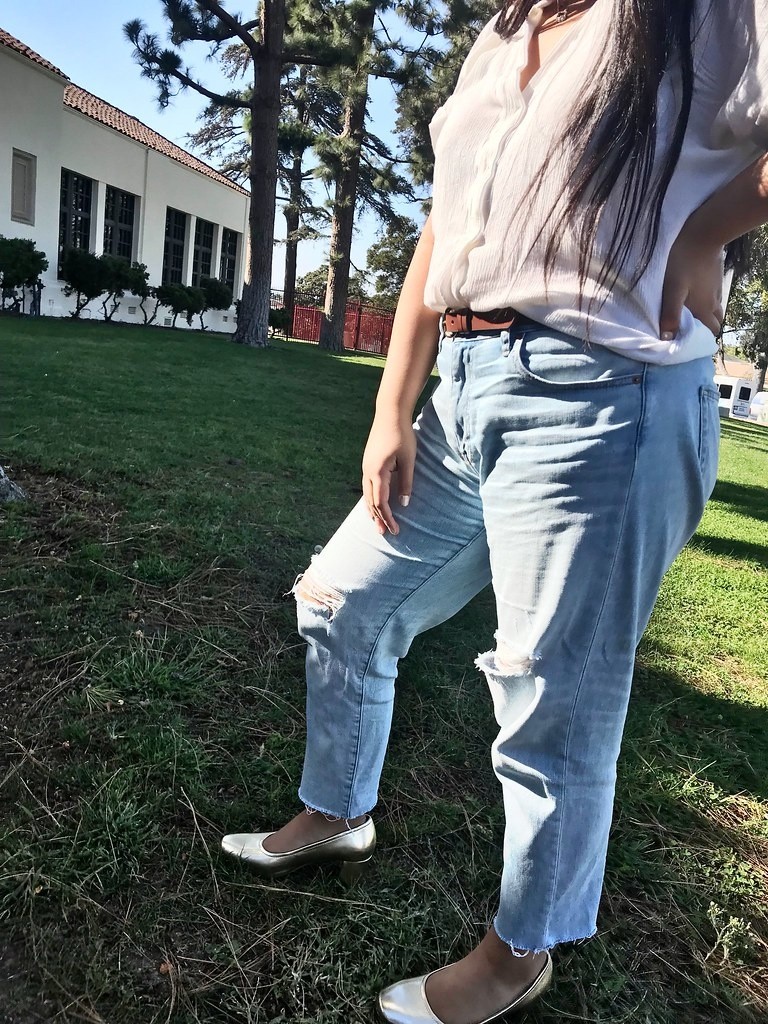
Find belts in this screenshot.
[440,305,533,335]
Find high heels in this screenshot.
[220,813,379,889]
[374,950,555,1024]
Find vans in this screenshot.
[712,374,756,422]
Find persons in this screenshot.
[222,1,768,1024]
